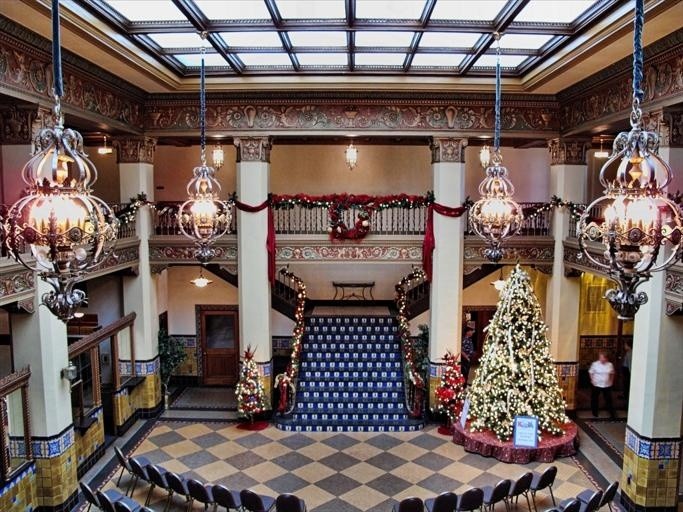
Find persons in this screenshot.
[618,339,632,401]
[462,327,474,388]
[588,351,622,421]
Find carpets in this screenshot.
[312,304,393,319]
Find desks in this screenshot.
[332,280,377,305]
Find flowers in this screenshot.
[267,190,438,245]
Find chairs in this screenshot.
[392,462,620,512]
[77,444,307,512]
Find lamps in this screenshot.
[61,360,81,387]
[344,138,359,171]
[476,137,494,170]
[572,0,681,323]
[172,28,237,263]
[466,30,526,263]
[3,0,124,324]
[209,142,226,172]
